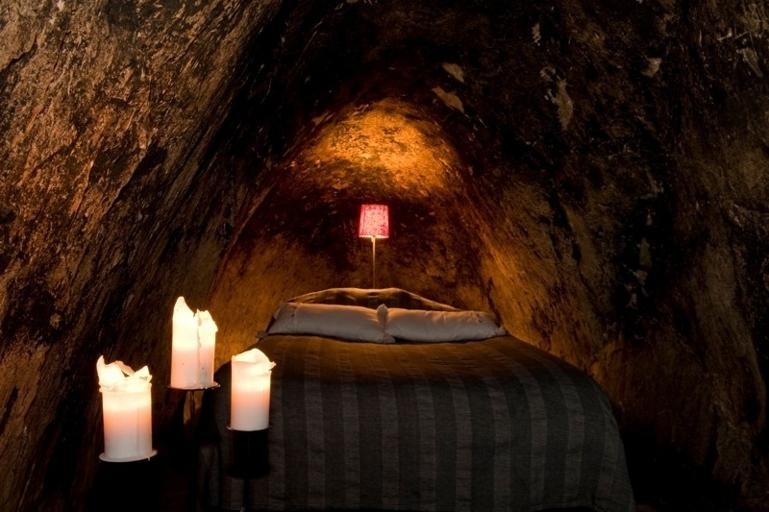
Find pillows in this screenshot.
[377,304,506,339]
[266,301,397,346]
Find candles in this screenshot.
[231,348,278,432]
[96,354,154,461]
[170,296,219,390]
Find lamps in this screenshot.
[358,204,390,289]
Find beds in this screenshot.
[185,287,637,512]
